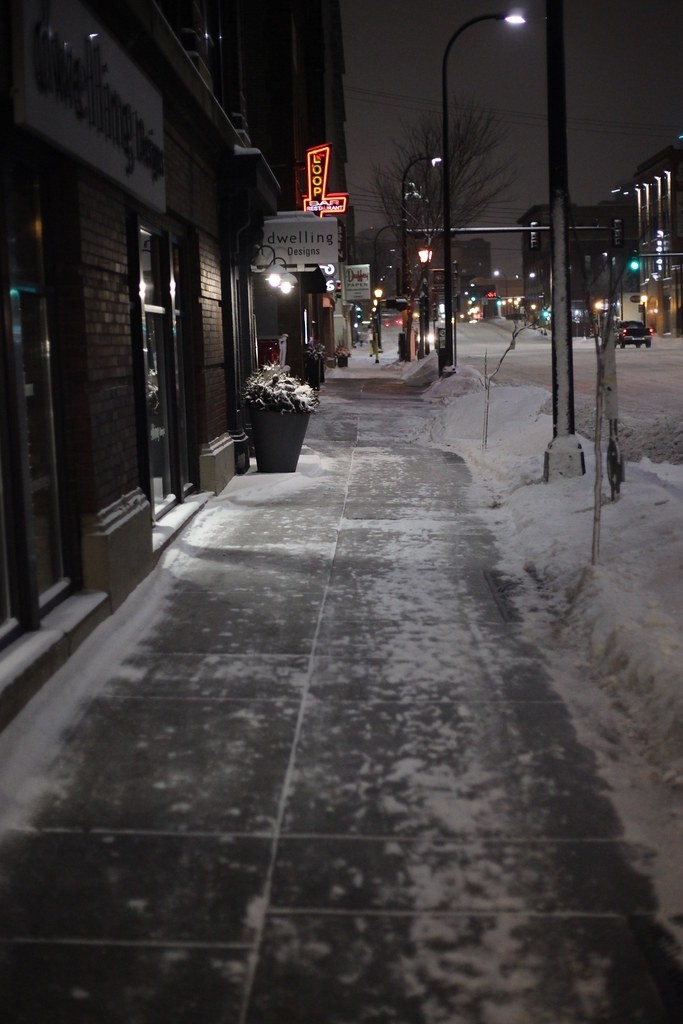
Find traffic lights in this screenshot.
[629,255,639,276]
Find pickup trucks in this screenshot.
[614,320,654,349]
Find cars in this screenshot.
[571,300,590,318]
[437,295,446,317]
[385,314,402,327]
[381,314,390,324]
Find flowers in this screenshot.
[336,344,351,357]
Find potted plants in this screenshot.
[243,360,319,473]
[307,344,326,382]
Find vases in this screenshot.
[337,357,348,368]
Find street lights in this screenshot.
[494,270,508,320]
[530,272,548,307]
[401,157,442,296]
[373,299,378,333]
[417,242,433,355]
[374,225,401,289]
[439,14,525,377]
[374,288,383,353]
[372,307,377,331]
[531,304,537,329]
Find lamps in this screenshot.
[247,244,287,290]
[250,256,297,295]
